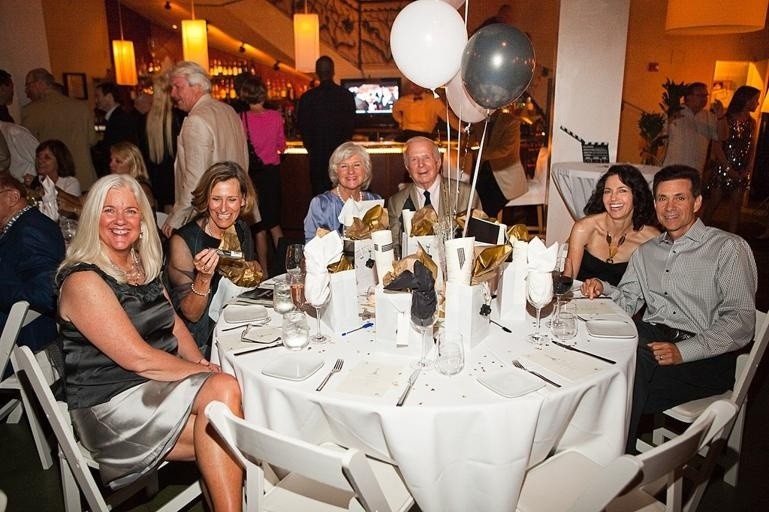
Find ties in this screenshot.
[423,192,431,208]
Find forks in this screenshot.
[513,360,563,389]
[315,359,346,391]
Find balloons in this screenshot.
[445,70,497,125]
[388,0,468,92]
[461,22,538,111]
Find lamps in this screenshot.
[183,20,210,77]
[666,0,768,36]
[112,40,139,86]
[294,13,320,73]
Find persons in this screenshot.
[0,120,41,182]
[145,65,188,213]
[0,174,67,355]
[392,80,464,141]
[58,140,157,223]
[461,108,530,218]
[238,74,289,281]
[52,173,246,512]
[703,84,762,234]
[563,164,662,286]
[298,55,357,196]
[90,80,147,177]
[22,138,82,200]
[168,161,261,346]
[161,60,262,241]
[579,163,759,454]
[387,136,483,259]
[0,69,16,125]
[303,142,384,240]
[19,68,103,195]
[662,81,730,174]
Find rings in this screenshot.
[660,355,663,361]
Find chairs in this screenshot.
[204,401,416,511]
[16,344,202,512]
[496,145,549,237]
[514,399,739,511]
[661,308,768,487]
[0,300,54,471]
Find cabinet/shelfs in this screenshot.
[285,141,461,237]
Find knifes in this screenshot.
[552,338,616,364]
[397,369,422,407]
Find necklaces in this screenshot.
[336,186,363,204]
[207,224,216,239]
[605,230,627,264]
[127,248,144,286]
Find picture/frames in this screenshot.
[63,73,88,100]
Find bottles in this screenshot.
[137,60,160,75]
[264,77,308,99]
[211,79,237,100]
[210,58,256,77]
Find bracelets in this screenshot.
[190,283,211,296]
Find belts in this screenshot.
[655,323,695,342]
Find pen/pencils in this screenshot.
[341,322,374,337]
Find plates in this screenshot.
[587,320,636,339]
[261,351,324,380]
[224,306,268,323]
[477,361,546,398]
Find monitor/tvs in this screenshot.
[340,77,401,124]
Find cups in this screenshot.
[526,272,554,338]
[285,244,304,279]
[304,271,333,343]
[274,283,294,328]
[550,301,575,344]
[290,272,305,310]
[434,332,465,375]
[409,291,439,372]
[282,311,310,379]
[545,257,573,329]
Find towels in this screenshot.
[303,230,343,306]
[328,256,352,272]
[218,227,263,286]
[382,260,437,324]
[456,208,503,235]
[507,224,530,241]
[526,237,558,306]
[339,200,384,226]
[411,205,439,235]
[346,204,389,240]
[473,244,512,278]
